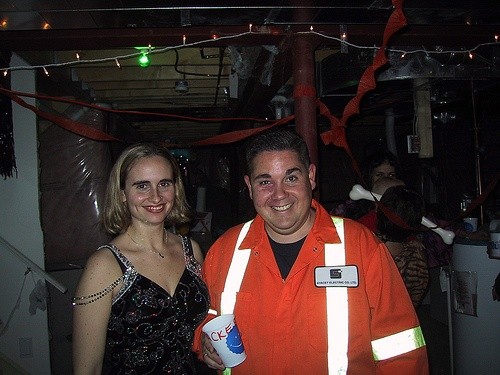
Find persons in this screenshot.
[72,143,210,375]
[192,130,429,375]
[329,149,490,308]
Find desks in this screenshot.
[448,238,500,375]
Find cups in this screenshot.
[490,233,500,257]
[464,218,478,232]
[203,313,248,367]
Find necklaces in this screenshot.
[126,228,168,259]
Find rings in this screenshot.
[203,353,208,359]
